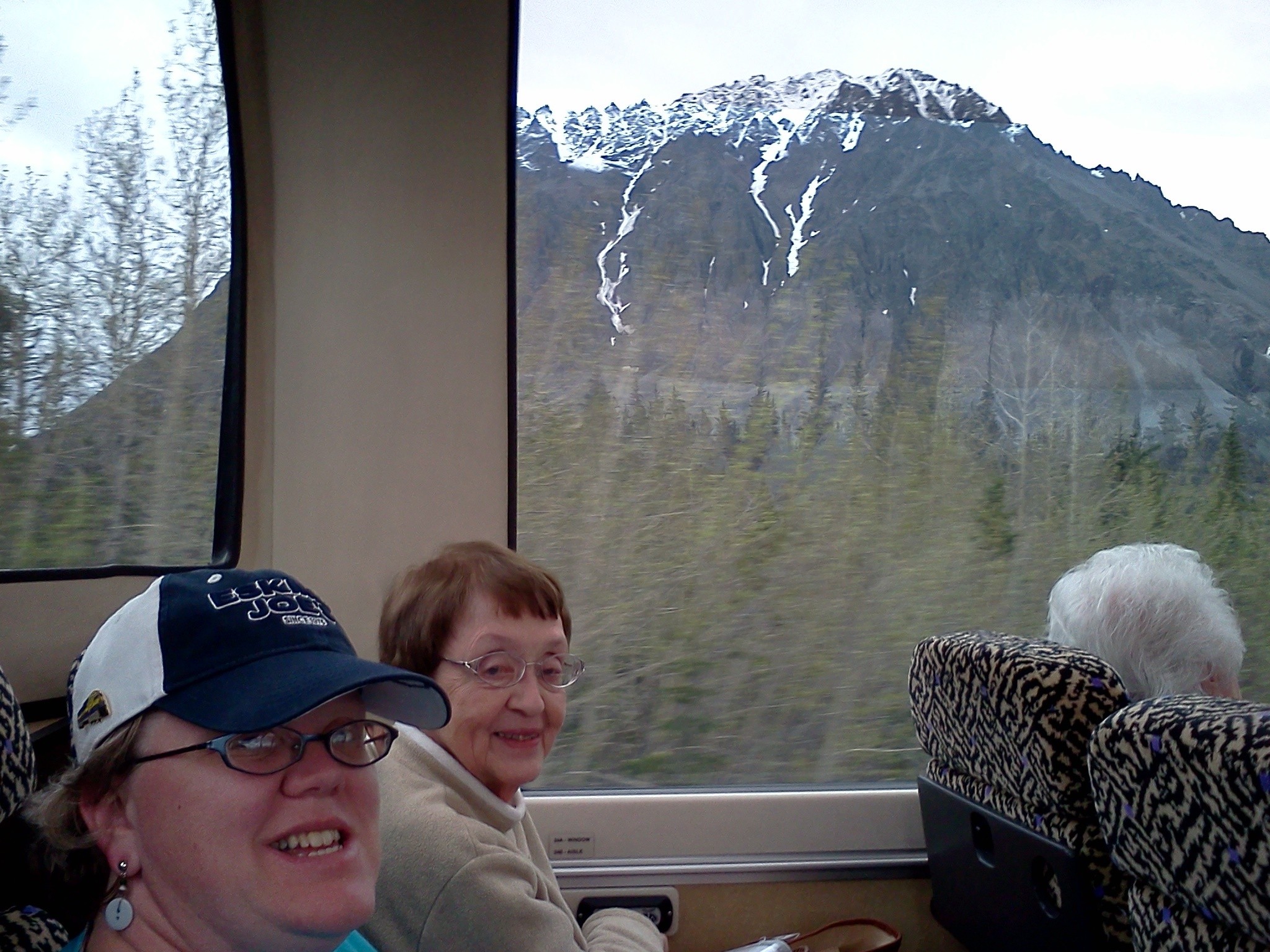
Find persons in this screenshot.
[351,532,674,952]
[1039,538,1250,707]
[20,561,453,952]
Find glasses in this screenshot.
[126,721,396,775]
[435,650,588,687]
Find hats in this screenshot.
[67,565,456,733]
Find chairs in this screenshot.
[906,629,1138,952]
[1088,694,1270,952]
